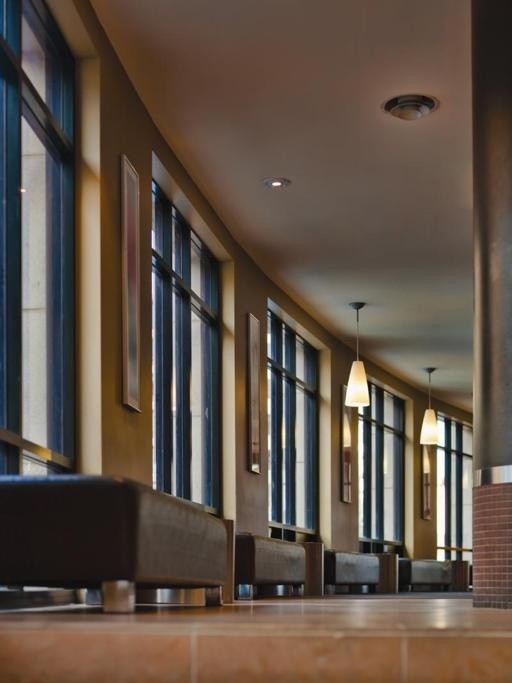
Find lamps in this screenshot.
[345,301,371,408]
[418,367,441,445]
[384,94,437,122]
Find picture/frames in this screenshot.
[120,155,142,415]
[341,385,352,504]
[245,313,264,477]
[421,445,433,520]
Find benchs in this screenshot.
[234,534,307,602]
[398,558,453,593]
[0,475,230,612]
[324,549,380,593]
